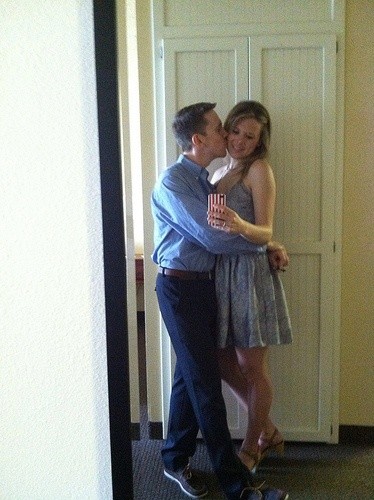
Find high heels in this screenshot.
[236,443,262,476]
[259,428,285,462]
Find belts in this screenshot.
[157,266,216,283]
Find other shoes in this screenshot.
[228,479,289,500]
[163,463,210,499]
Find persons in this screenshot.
[208,100,294,474]
[151,102,290,500]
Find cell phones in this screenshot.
[207,194,227,226]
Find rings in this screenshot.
[222,222,226,227]
